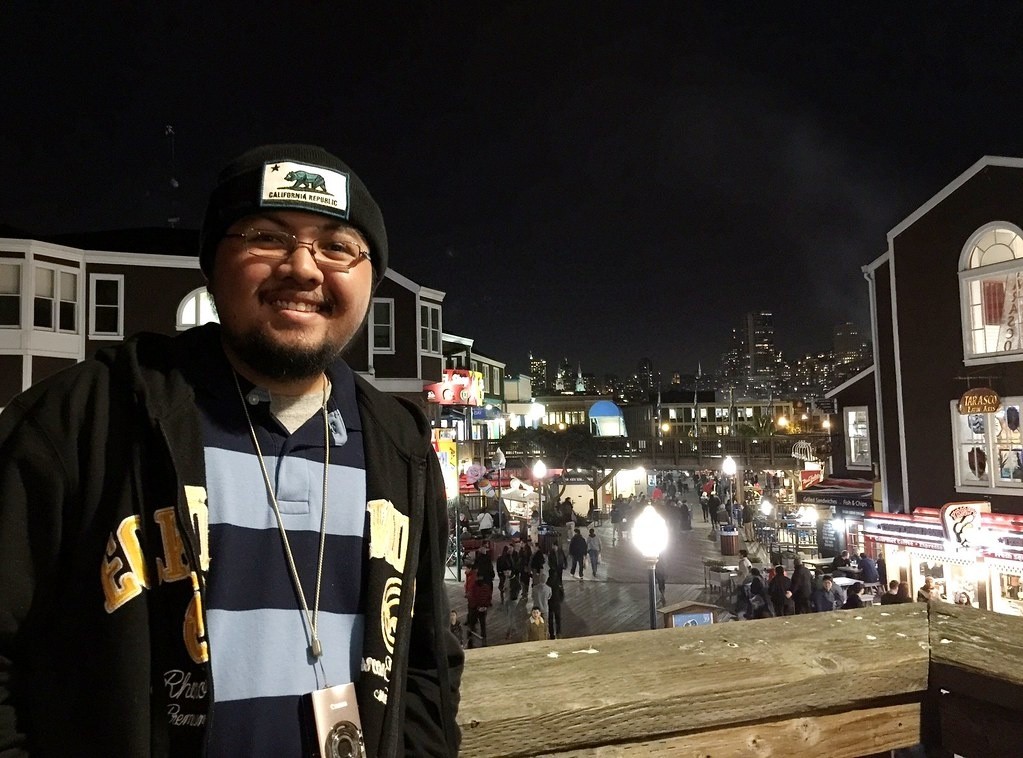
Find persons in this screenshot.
[569,529,587,578]
[743,470,778,489]
[955,592,971,604]
[840,583,864,610]
[463,541,567,642]
[611,473,739,542]
[880,580,898,605]
[587,529,602,576]
[785,576,842,612]
[749,569,768,596]
[0,140,464,758]
[893,583,912,603]
[560,497,573,520]
[876,552,886,589]
[790,559,812,606]
[744,502,754,542]
[918,577,939,601]
[477,508,494,534]
[450,611,462,645]
[458,514,471,539]
[768,566,791,615]
[831,550,849,577]
[857,553,877,583]
[730,550,752,614]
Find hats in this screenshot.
[199,143,389,290]
[702,492,707,496]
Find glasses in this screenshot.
[225,227,372,270]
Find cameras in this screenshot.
[302,682,368,758]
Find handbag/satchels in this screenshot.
[584,557,588,569]
[597,553,602,564]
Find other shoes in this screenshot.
[706,519,709,523]
[752,539,755,542]
[728,610,738,617]
[704,519,706,523]
[744,540,750,542]
[743,614,751,620]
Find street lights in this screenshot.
[722,456,736,528]
[491,446,507,528]
[632,504,670,629]
[532,459,547,533]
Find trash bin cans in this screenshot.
[537,525,561,550]
[719,525,739,555]
